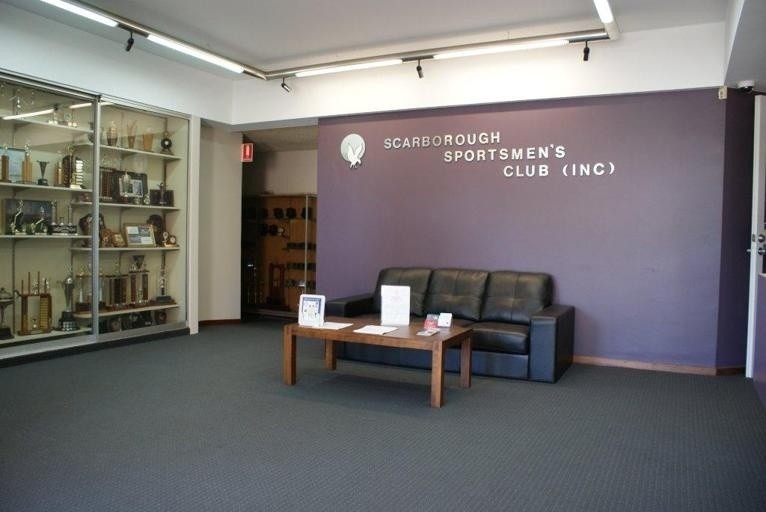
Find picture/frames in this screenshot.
[121,221,157,249]
[111,169,149,206]
[96,165,119,203]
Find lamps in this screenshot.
[48,0,119,29]
[280,77,293,93]
[3,98,112,126]
[147,34,246,75]
[293,58,405,80]
[416,58,424,78]
[126,32,136,52]
[432,39,570,60]
[584,40,590,61]
[594,1,620,43]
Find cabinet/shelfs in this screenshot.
[68,94,183,349]
[0,71,94,367]
[241,192,317,322]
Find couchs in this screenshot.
[324,265,581,388]
